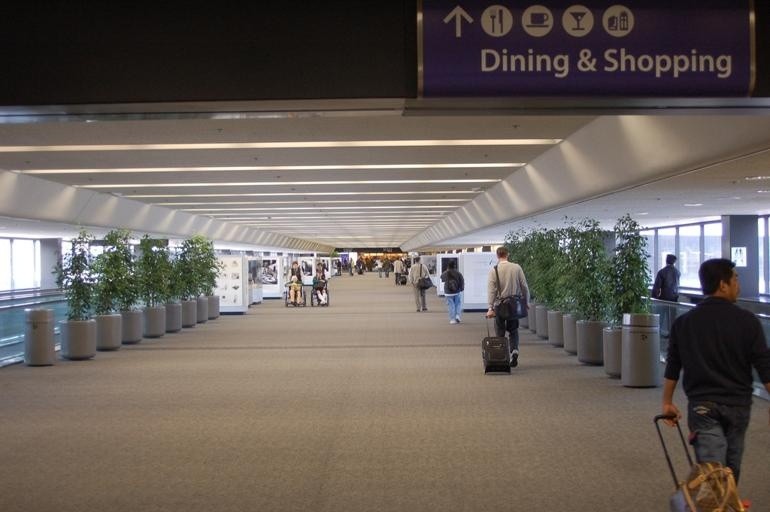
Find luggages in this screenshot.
[400,276,406,285]
[482,337,511,374]
[653,414,745,512]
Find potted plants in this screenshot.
[55,225,224,363]
[496,212,653,380]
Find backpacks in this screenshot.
[448,270,458,291]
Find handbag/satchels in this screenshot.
[418,277,432,289]
[496,295,527,319]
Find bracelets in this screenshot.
[488,307,494,311]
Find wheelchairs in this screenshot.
[310,285,330,306]
[282,279,307,308]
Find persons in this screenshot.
[284,275,305,305]
[322,254,409,286]
[485,246,532,369]
[312,272,328,305]
[287,261,303,299]
[661,256,769,491]
[315,263,329,289]
[408,257,430,312]
[439,260,465,324]
[651,252,683,331]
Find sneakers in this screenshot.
[416,308,428,313]
[449,315,462,324]
[511,350,519,367]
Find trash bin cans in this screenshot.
[621,313,660,387]
[24,308,56,366]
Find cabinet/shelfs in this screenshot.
[213,252,343,316]
[406,249,500,313]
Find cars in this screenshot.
[250,265,278,285]
